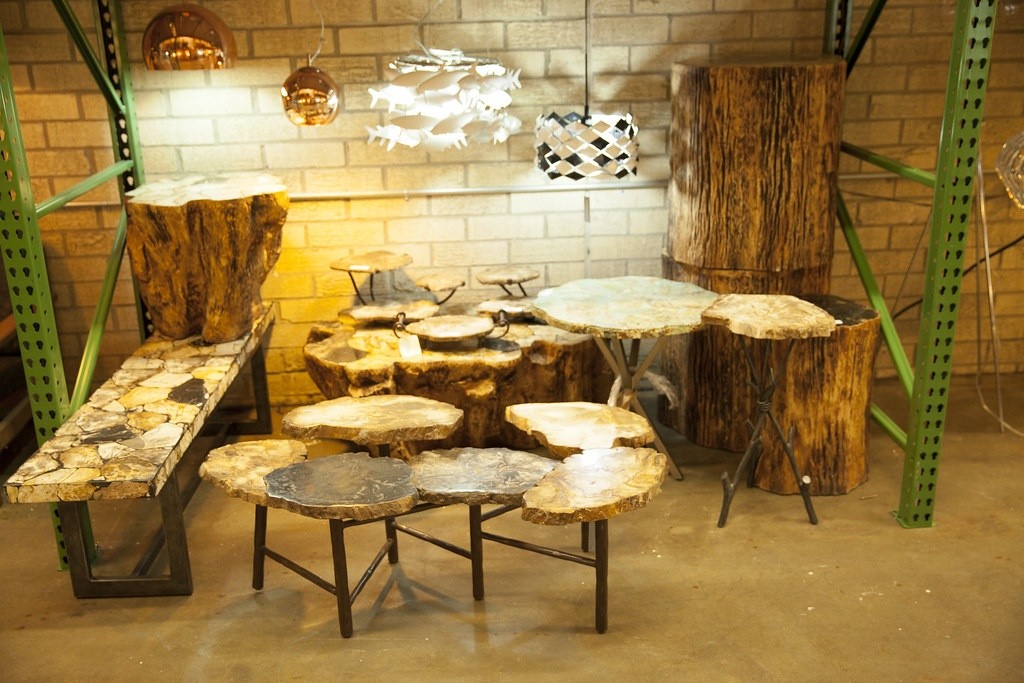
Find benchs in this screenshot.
[5,302,275,600]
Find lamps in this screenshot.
[140,0,238,70]
[280,0,339,125]
[535,0,637,181]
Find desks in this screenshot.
[540,277,718,478]
[703,294,838,522]
[204,394,665,639]
